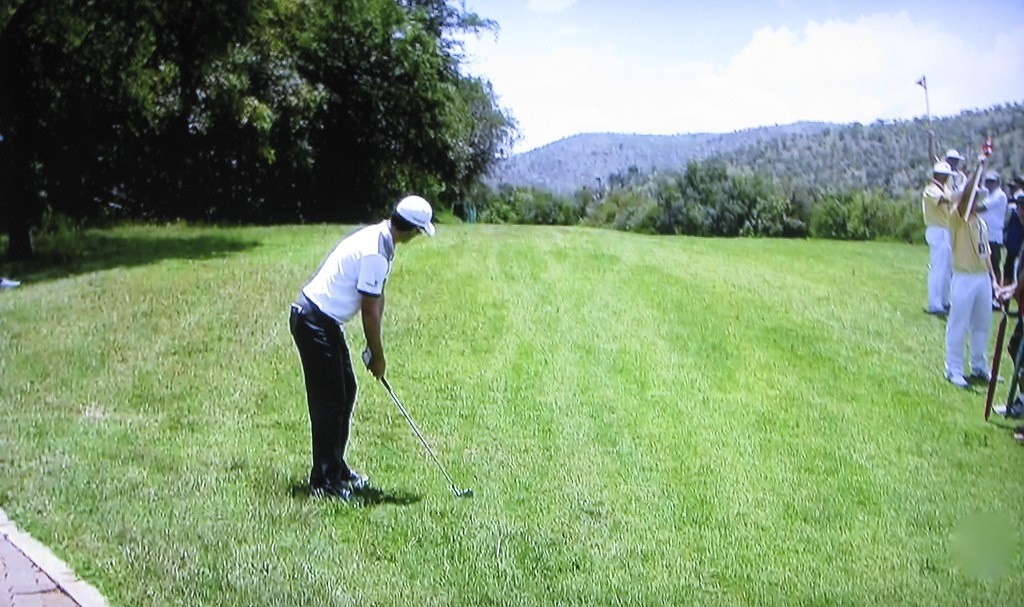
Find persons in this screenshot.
[922,128,1024,441]
[288,195,435,493]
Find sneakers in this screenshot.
[342,471,371,490]
[310,476,354,501]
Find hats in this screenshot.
[933,161,957,176]
[946,149,965,162]
[395,194,435,237]
[984,171,999,182]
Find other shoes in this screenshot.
[935,306,948,315]
[971,368,1003,383]
[943,368,968,386]
[992,397,1024,418]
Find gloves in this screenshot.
[362,347,373,366]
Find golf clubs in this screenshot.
[361,351,475,497]
[962,131,997,223]
